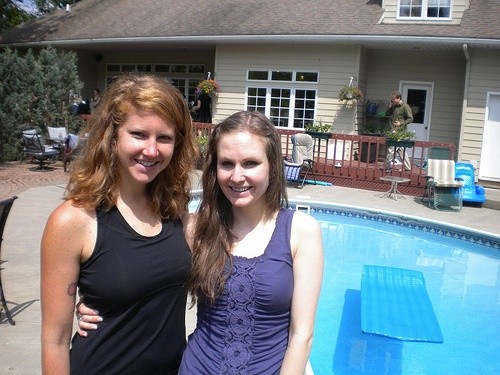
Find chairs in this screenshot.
[282,133,317,189]
[0,196,19,326]
[20,127,80,171]
[422,146,465,212]
[455,163,486,208]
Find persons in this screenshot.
[40,74,200,375]
[91,88,101,109]
[385,91,413,172]
[76,111,324,375]
[69,93,86,115]
[192,92,211,122]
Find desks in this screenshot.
[45,137,65,161]
[380,175,411,201]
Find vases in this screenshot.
[387,138,413,148]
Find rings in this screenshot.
[78,314,82,319]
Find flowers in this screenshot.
[195,79,221,95]
[385,128,416,142]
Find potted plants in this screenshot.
[305,121,330,139]
[362,127,385,145]
[339,86,363,101]
